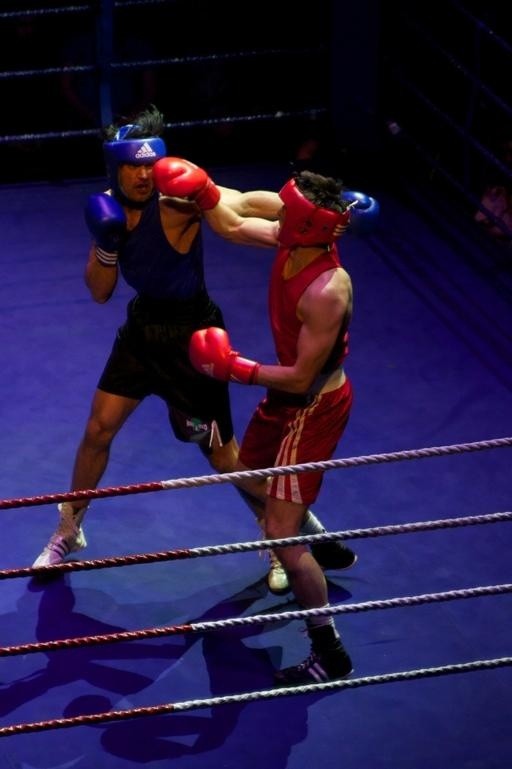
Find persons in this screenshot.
[31,103,383,594]
[152,154,356,689]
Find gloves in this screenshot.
[189,327,260,385]
[342,191,380,220]
[152,157,220,210]
[84,192,127,266]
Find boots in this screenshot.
[308,541,357,571]
[275,626,354,687]
[32,502,87,569]
[257,529,290,590]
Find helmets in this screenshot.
[276,177,352,247]
[103,124,167,202]
